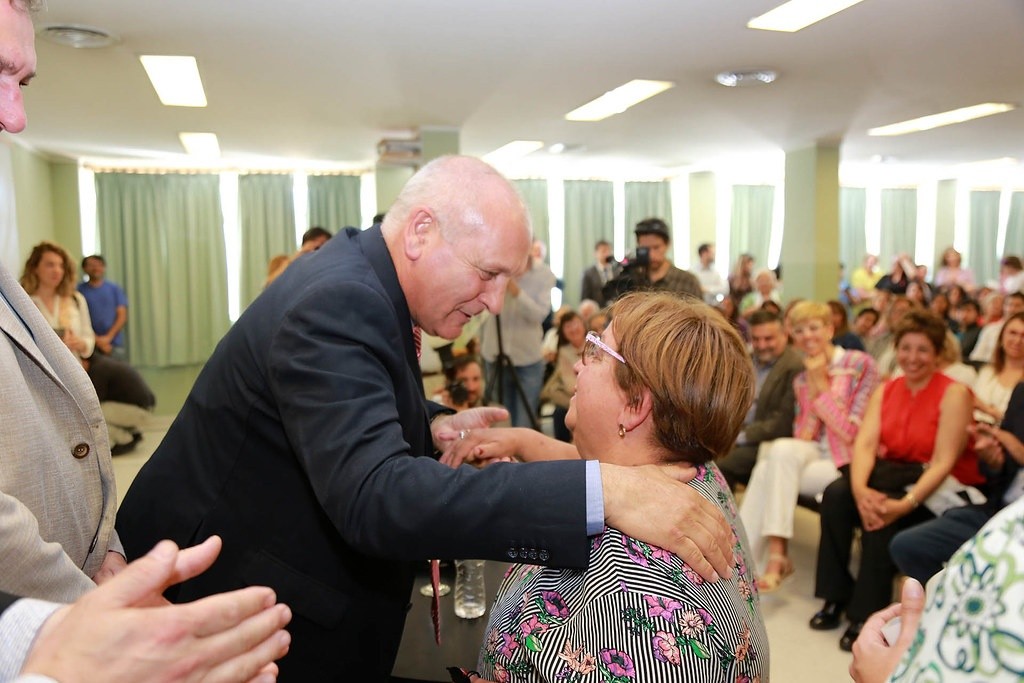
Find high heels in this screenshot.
[811,598,849,631]
[840,620,867,652]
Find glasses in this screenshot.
[582,330,633,367]
[635,223,668,238]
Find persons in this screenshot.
[18,239,96,369]
[0,0,292,683]
[264,212,882,588]
[113,151,738,683]
[808,246,1024,683]
[76,253,128,361]
[437,287,771,682]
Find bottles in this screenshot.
[453,559,486,619]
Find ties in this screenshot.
[413,325,422,364]
[604,268,609,282]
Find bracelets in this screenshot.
[906,493,919,509]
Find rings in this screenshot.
[460,430,465,441]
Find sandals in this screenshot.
[757,553,796,593]
